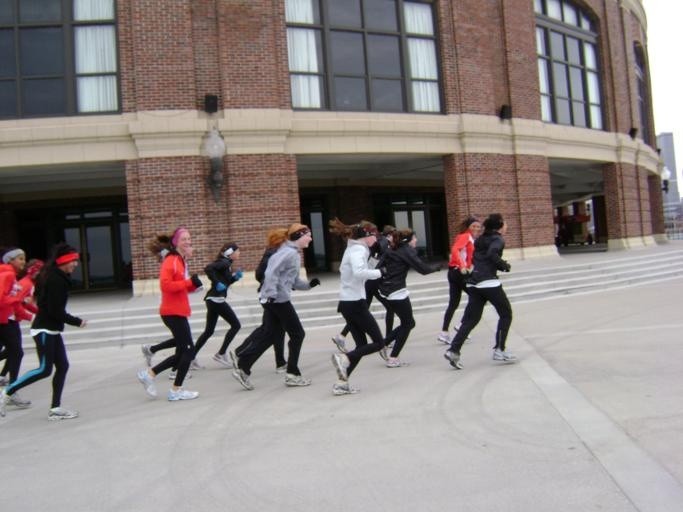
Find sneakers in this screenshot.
[168,387,199,401]
[332,332,348,354]
[232,368,253,390]
[168,368,189,379]
[0,375,77,420]
[444,350,463,371]
[492,349,515,362]
[275,363,288,374]
[387,357,406,368]
[230,350,239,369]
[331,353,350,381]
[332,383,361,395]
[142,344,154,367]
[212,352,231,366]
[284,373,311,386]
[454,323,460,332]
[438,332,452,344]
[192,359,201,369]
[138,369,158,399]
[379,345,388,361]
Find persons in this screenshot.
[190,241,243,368]
[226,222,320,390]
[436,214,518,370]
[0,243,87,420]
[326,218,444,394]
[135,226,200,401]
